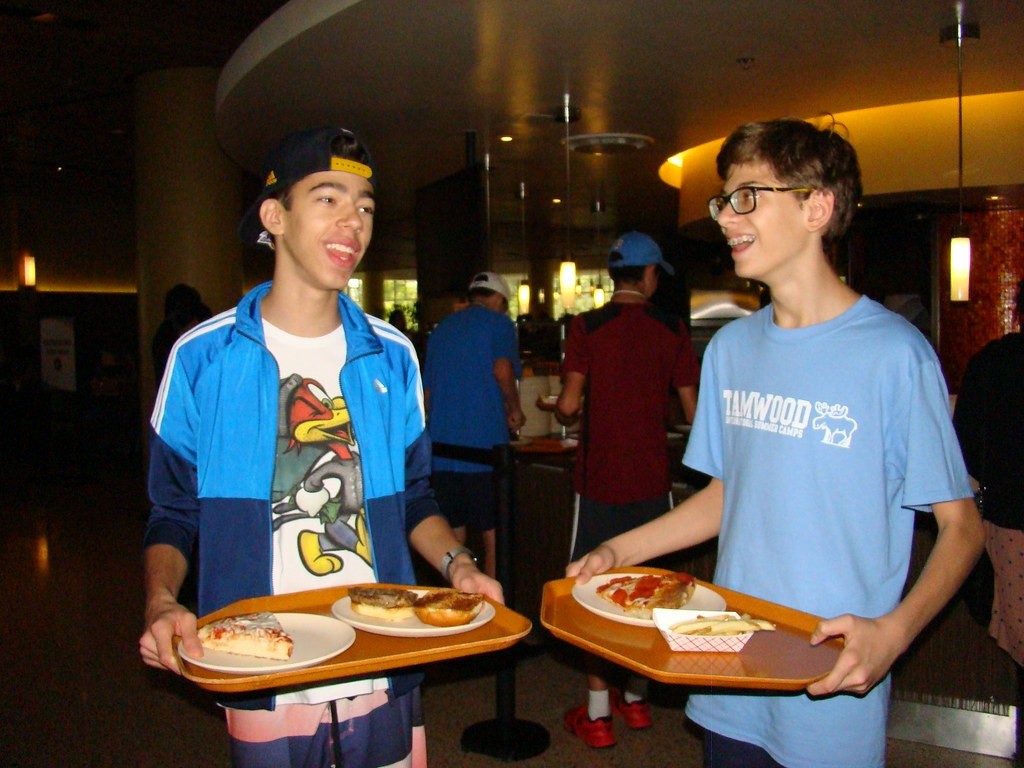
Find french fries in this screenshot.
[671,613,777,636]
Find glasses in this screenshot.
[708,186,811,221]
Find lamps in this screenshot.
[939,23,981,302]
[516,91,605,315]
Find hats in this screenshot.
[468,272,512,317]
[605,231,675,275]
[237,124,384,259]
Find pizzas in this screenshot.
[198,611,294,660]
[596,572,696,620]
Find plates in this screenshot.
[667,432,685,439]
[570,572,728,627]
[330,590,497,638]
[513,373,585,436]
[176,613,357,676]
[510,437,532,446]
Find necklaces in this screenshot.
[472,300,487,308]
[611,289,645,298]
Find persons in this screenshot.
[567,117,987,768]
[149,229,699,749]
[140,126,525,768]
[949,276,1024,768]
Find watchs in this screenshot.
[441,547,478,583]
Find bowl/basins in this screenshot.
[674,425,691,431]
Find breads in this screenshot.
[412,591,484,626]
[348,586,418,620]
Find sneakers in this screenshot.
[608,686,653,727]
[560,703,618,747]
